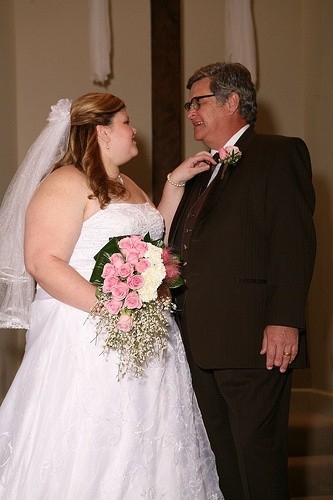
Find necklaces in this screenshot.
[110,174,125,198]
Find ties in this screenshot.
[198,153,220,198]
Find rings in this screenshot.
[282,352,290,356]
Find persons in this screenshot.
[1,94,228,500]
[164,63,316,499]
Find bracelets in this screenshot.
[166,173,186,187]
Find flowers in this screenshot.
[216,146,242,178]
[84,232,186,382]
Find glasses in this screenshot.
[184,94,215,112]
